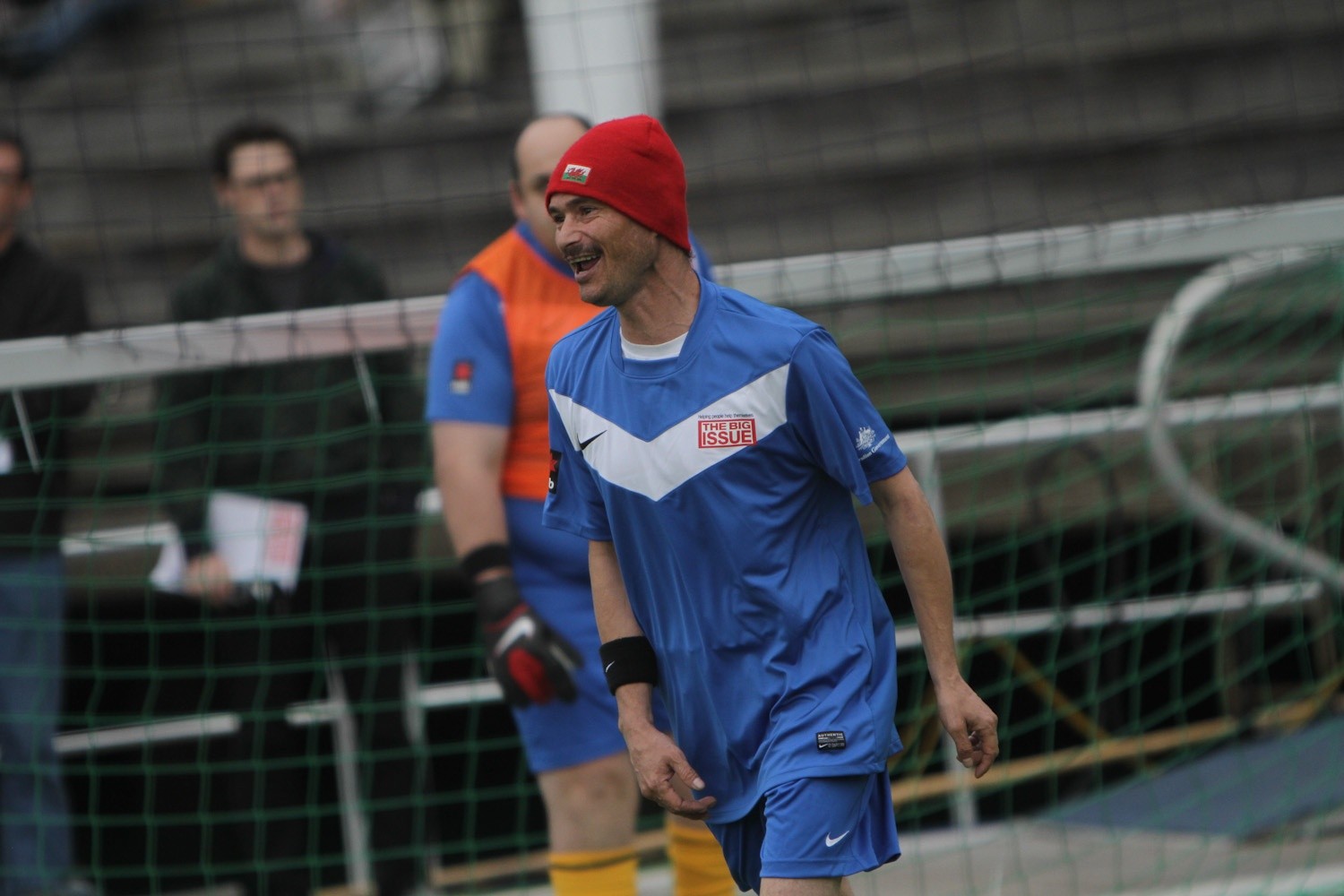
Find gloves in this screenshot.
[471,578,587,710]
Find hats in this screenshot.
[543,114,691,254]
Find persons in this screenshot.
[538,113,1001,896]
[421,108,710,896]
[0,126,103,896]
[159,114,433,896]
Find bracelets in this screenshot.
[600,635,660,696]
